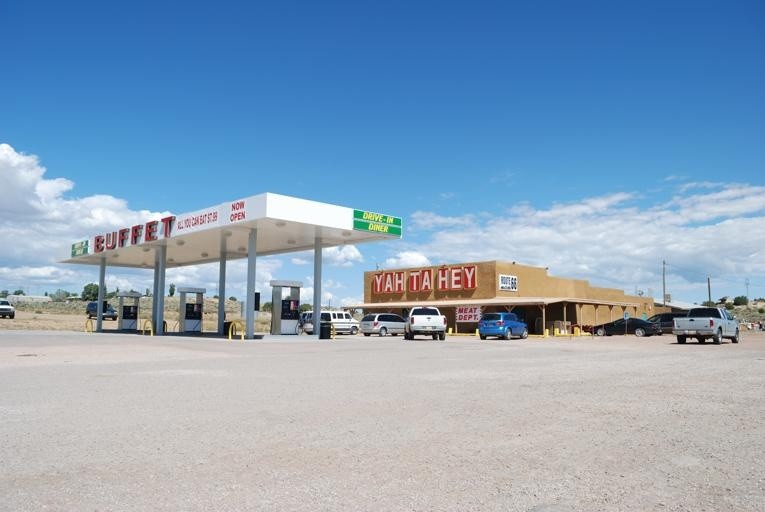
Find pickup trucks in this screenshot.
[403,306,448,340]
[672,307,740,344]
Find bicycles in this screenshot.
[295,319,305,335]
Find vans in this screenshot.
[301,310,359,335]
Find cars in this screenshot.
[590,312,687,336]
[478,312,528,340]
[86,301,118,320]
[0,300,15,319]
[359,312,407,337]
